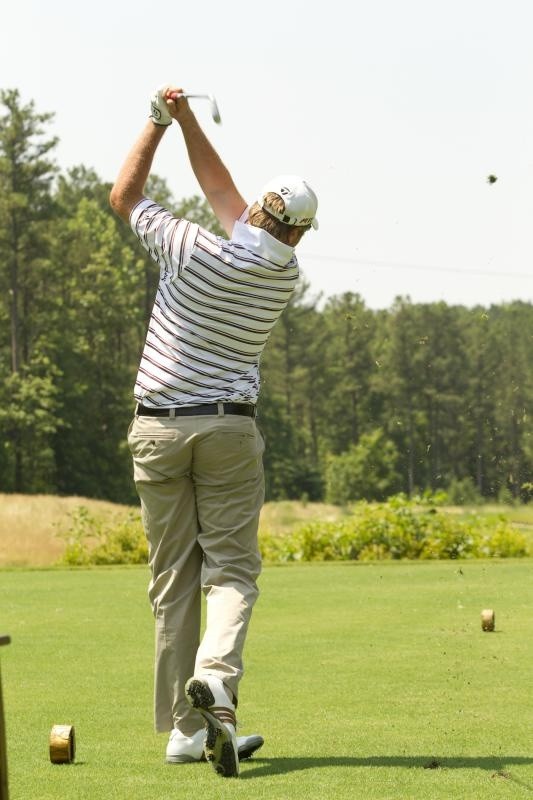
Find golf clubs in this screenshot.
[170,92,222,124]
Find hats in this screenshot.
[257,175,319,231]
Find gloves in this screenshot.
[148,84,172,126]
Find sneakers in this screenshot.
[184,674,240,778]
[167,727,263,762]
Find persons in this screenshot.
[109,86,318,778]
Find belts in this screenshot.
[137,402,255,417]
